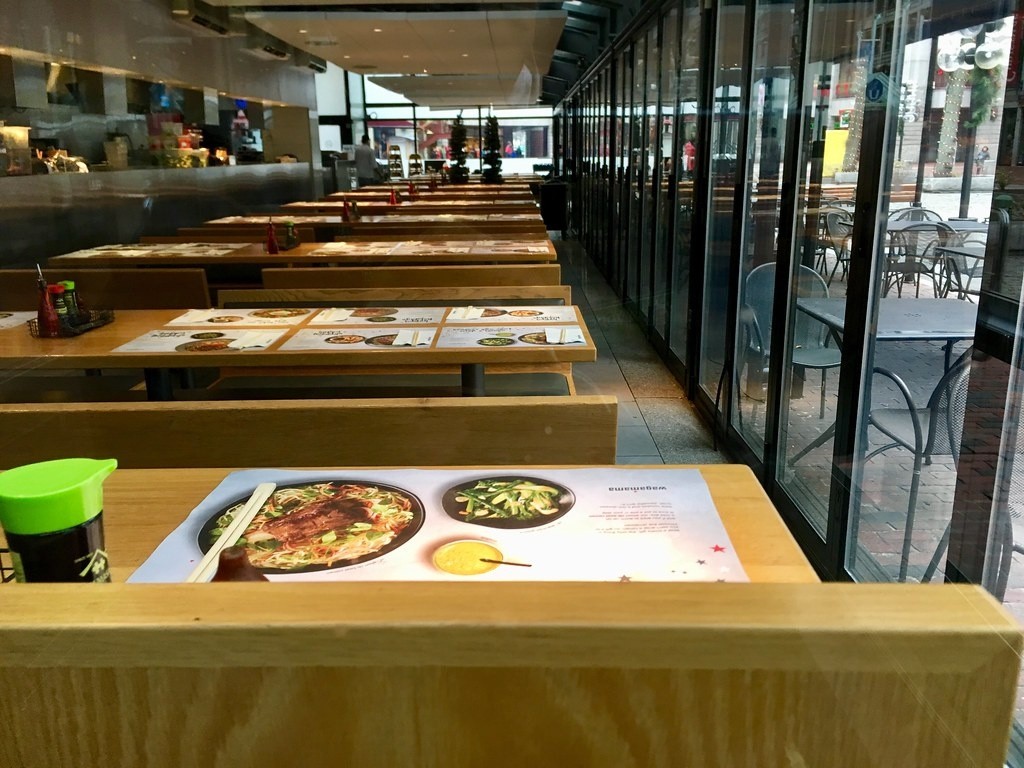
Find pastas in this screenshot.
[209,482,414,569]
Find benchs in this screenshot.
[1,269,212,312]
[216,287,578,400]
[261,267,562,288]
[1,394,620,475]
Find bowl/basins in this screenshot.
[429,539,505,577]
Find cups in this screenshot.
[0,456,118,584]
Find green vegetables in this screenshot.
[481,338,512,346]
[454,478,562,521]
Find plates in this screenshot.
[175,339,237,351]
[248,307,310,319]
[324,334,365,345]
[441,476,575,529]
[509,310,544,317]
[208,315,244,325]
[368,316,396,322]
[196,479,426,574]
[481,308,508,317]
[518,332,563,345]
[190,332,225,339]
[348,308,399,318]
[366,334,400,345]
[476,337,515,346]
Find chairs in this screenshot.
[634,175,1024,581]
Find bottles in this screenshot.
[390,189,397,205]
[283,220,297,250]
[36,275,60,339]
[46,285,72,329]
[407,171,448,194]
[394,190,402,204]
[263,214,279,255]
[59,281,82,323]
[340,195,350,222]
[351,200,360,220]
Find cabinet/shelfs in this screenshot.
[408,153,423,178]
[387,145,405,178]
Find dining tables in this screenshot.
[46,173,556,265]
[0,304,598,403]
[1,464,828,583]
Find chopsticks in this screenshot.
[184,482,276,583]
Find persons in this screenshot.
[355,135,383,186]
[752,127,780,173]
[505,141,512,154]
[976,146,990,174]
[686,137,695,172]
